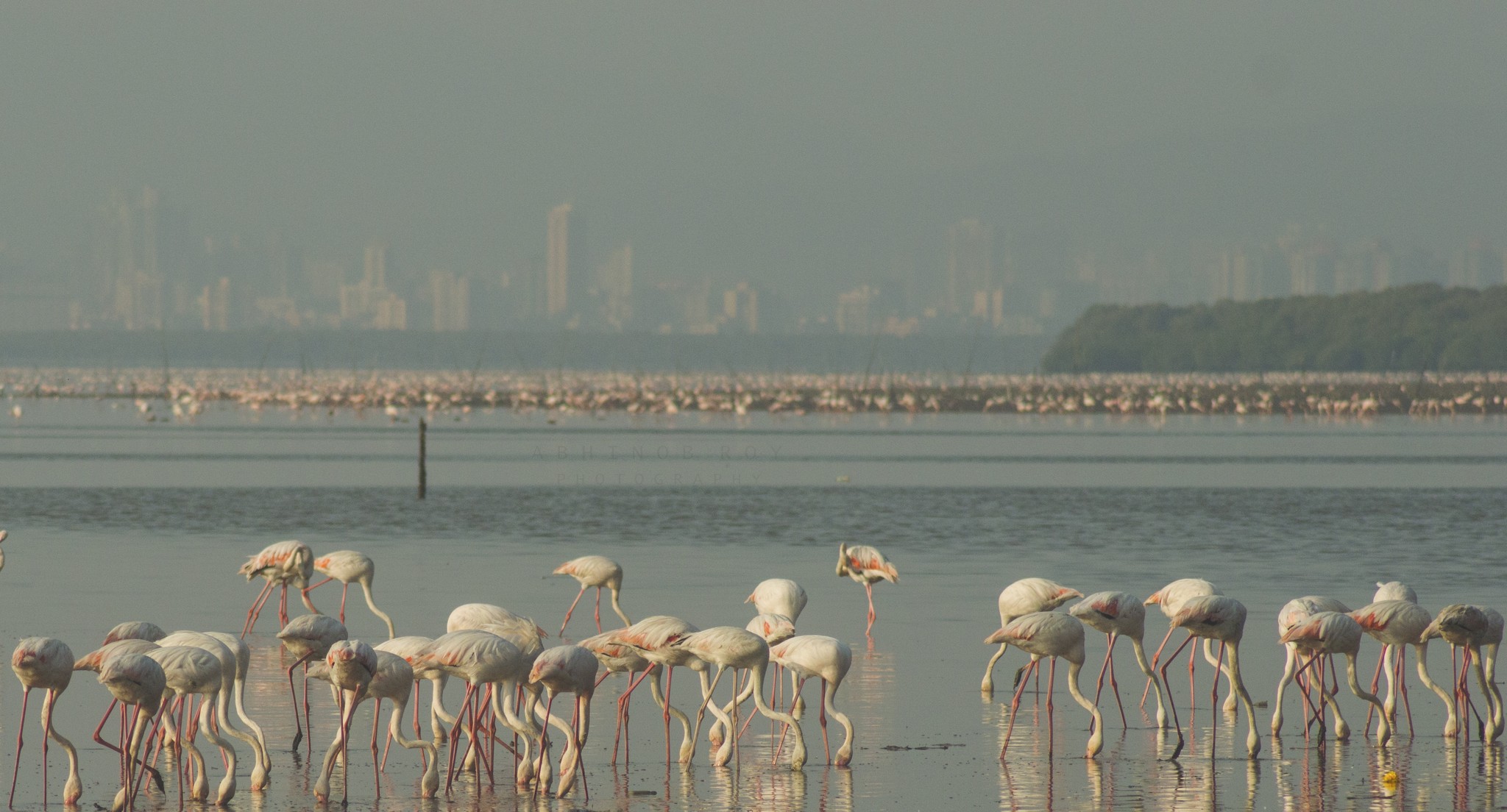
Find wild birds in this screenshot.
[1159,593,1262,761]
[69,577,857,811]
[985,611,1105,763]
[834,540,902,649]
[237,538,396,645]
[979,576,1087,706]
[1268,580,1505,747]
[550,555,632,640]
[8,636,84,812]
[0,529,9,572]
[1141,577,1239,726]
[1070,590,1170,731]
[1,362,1506,429]
